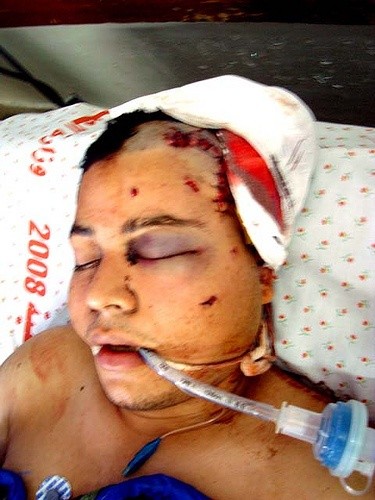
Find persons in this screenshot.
[0,108,375,499]
